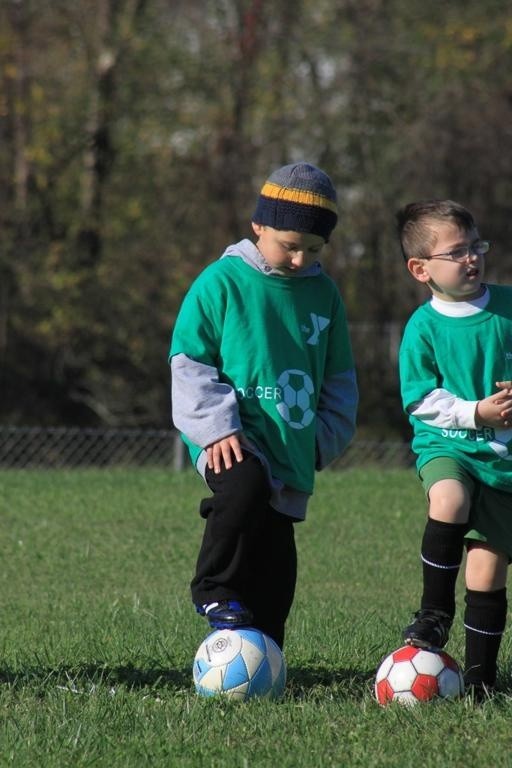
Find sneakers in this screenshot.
[193,598,256,631]
[403,606,456,652]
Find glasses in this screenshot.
[417,237,492,265]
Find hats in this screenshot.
[249,162,340,242]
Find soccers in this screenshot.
[375,645,465,709]
[193,628,286,704]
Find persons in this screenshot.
[168,161,362,648]
[388,201,511,702]
